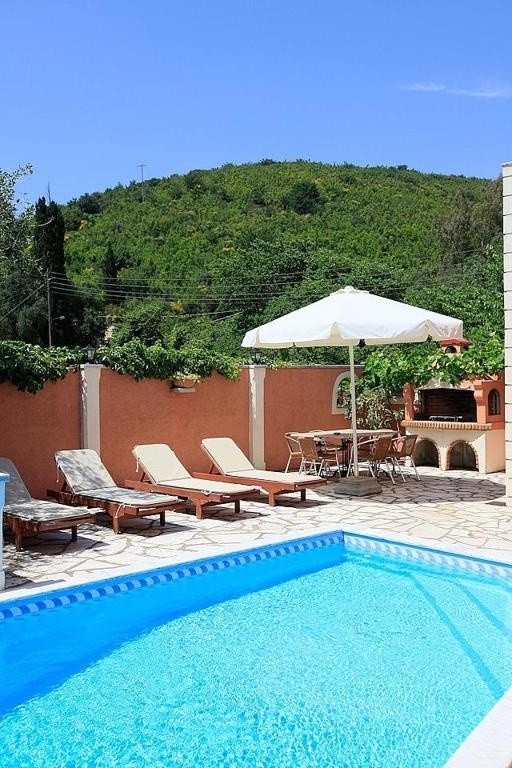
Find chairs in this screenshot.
[283,428,417,486]
[191,437,328,507]
[1,455,97,552]
[124,442,261,520]
[45,446,186,536]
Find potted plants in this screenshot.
[170,371,200,388]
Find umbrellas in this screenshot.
[241,286,463,477]
